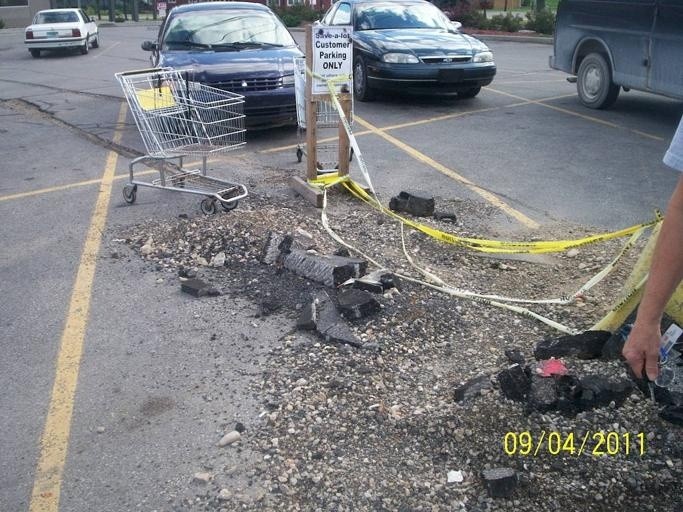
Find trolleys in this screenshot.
[115,67,248,215]
[293,57,354,172]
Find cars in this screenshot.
[320,1,496,101]
[24,8,99,57]
[141,1,306,142]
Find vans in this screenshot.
[549,0,683,109]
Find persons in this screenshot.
[623,112,683,382]
